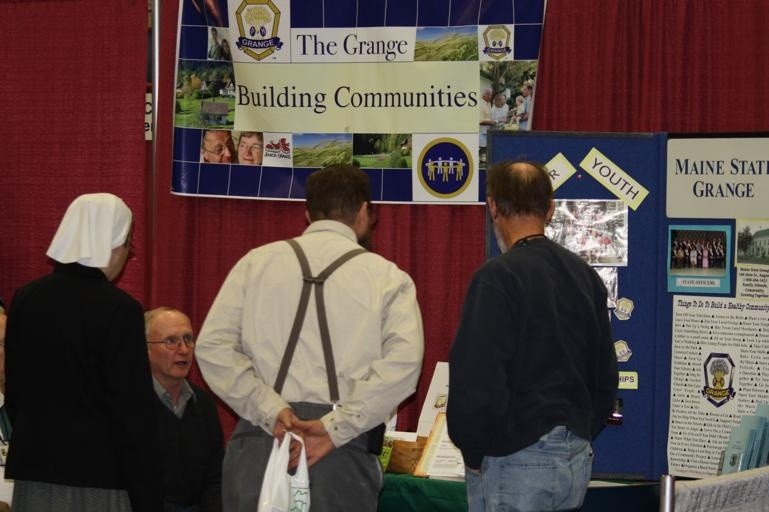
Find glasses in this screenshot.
[146,333,194,350]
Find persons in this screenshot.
[4,192,163,511]
[442,153,618,512]
[671,236,727,269]
[513,79,533,131]
[236,130,264,165]
[195,161,427,511]
[219,39,232,61]
[0,305,13,472]
[491,93,510,124]
[138,307,227,511]
[202,128,236,163]
[511,95,526,127]
[479,86,496,165]
[206,28,221,61]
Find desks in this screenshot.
[379,473,662,512]
